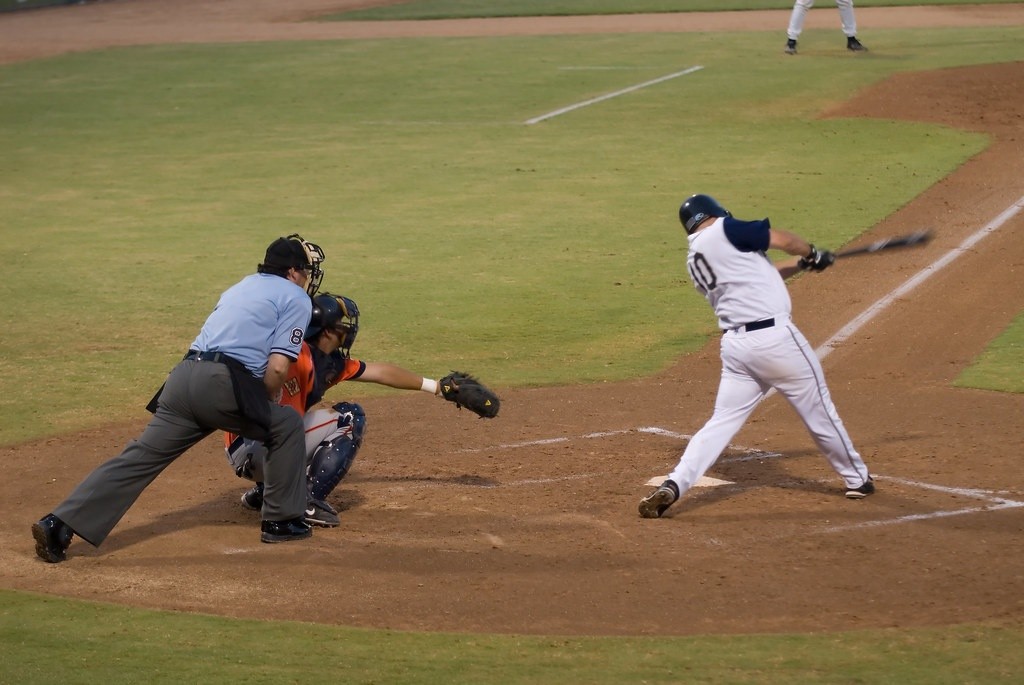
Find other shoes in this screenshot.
[848,40,862,49]
[784,38,797,55]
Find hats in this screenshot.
[263,237,310,270]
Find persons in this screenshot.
[785,0,867,55]
[225,292,499,525]
[31,234,326,563]
[638,194,875,519]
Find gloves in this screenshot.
[235,453,253,478]
[797,244,833,272]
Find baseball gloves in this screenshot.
[439,368,505,423]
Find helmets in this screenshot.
[303,295,344,340]
[679,195,732,235]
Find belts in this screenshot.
[723,318,774,334]
[228,436,244,455]
[185,351,222,364]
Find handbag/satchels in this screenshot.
[223,355,273,440]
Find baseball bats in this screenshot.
[800,233,928,264]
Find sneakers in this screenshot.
[32,513,74,563]
[638,480,679,518]
[260,517,312,543]
[241,485,263,510]
[304,497,340,528]
[845,474,874,497]
